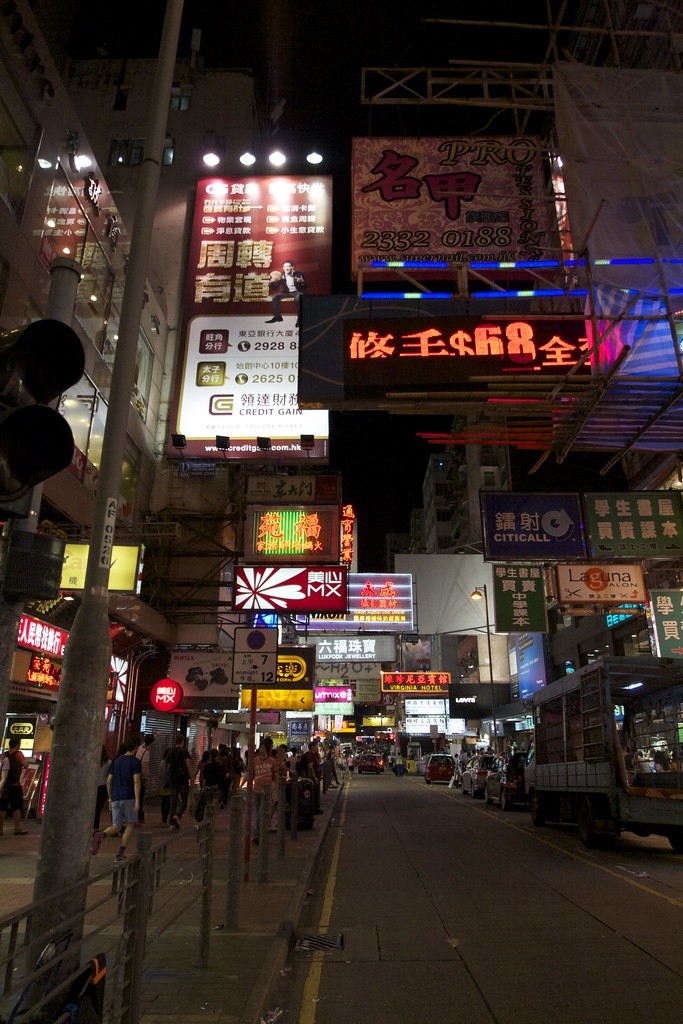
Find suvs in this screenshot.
[459,751,527,810]
[357,754,385,774]
[425,754,456,783]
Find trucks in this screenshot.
[526,654,683,853]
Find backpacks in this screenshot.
[301,752,314,770]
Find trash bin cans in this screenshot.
[287,778,316,830]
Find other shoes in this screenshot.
[269,825,278,832]
[252,836,260,844]
[114,856,126,863]
[14,828,27,836]
[161,822,169,827]
[170,818,181,831]
[91,832,102,855]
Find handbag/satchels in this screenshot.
[157,754,171,780]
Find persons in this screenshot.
[633,746,683,773]
[0,737,30,835]
[384,746,494,776]
[264,261,308,327]
[91,734,354,861]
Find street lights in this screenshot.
[470,584,497,754]
